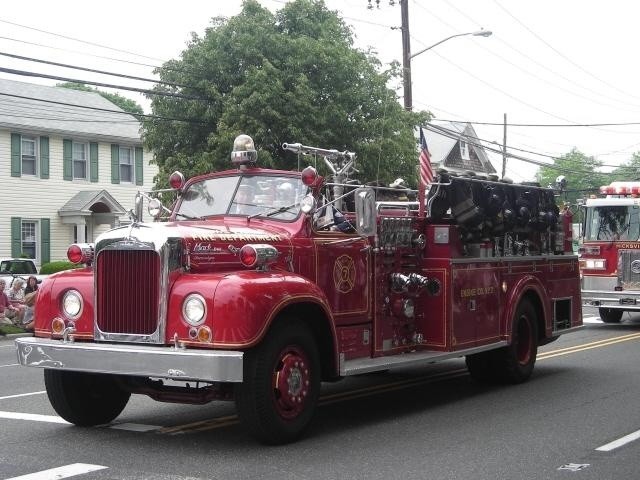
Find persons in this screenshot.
[23,276,40,328]
[6,278,29,324]
[228,179,259,214]
[0,279,19,324]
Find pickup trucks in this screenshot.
[0,258,48,289]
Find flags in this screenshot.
[420,126,434,186]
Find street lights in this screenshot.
[401,27,492,111]
